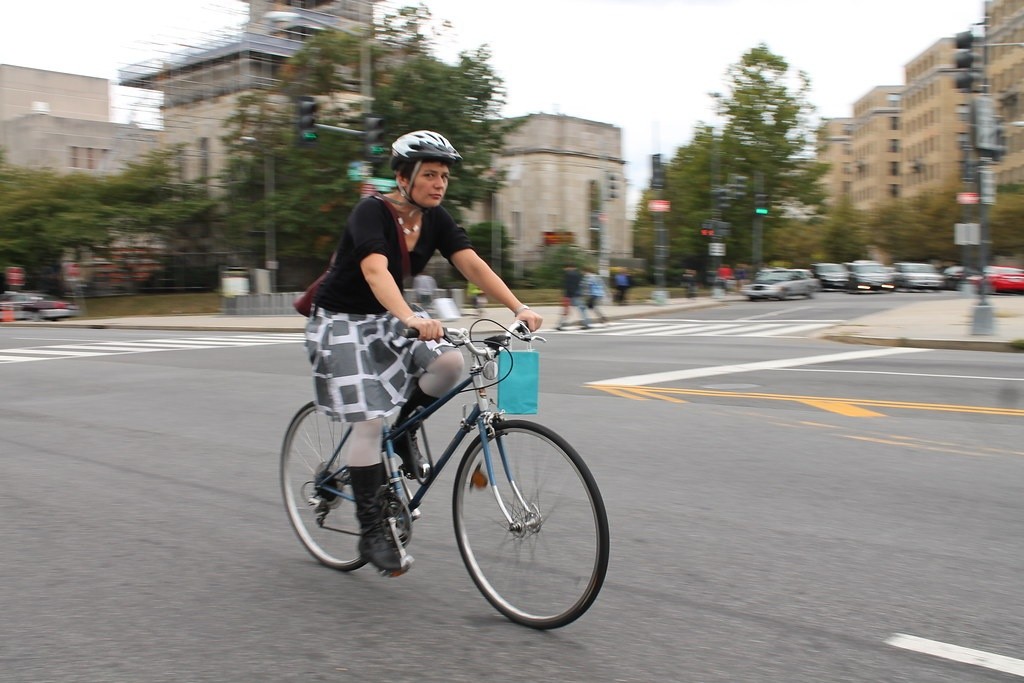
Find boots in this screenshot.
[388,422,428,477]
[358,483,414,570]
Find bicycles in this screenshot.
[280,311,614,632]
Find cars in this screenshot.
[942,264,1024,295]
[889,260,947,292]
[813,261,852,291]
[744,270,823,302]
[846,258,898,295]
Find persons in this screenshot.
[304,129,543,571]
[717,264,735,280]
[557,267,608,331]
[615,275,628,300]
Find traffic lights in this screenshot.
[717,187,734,213]
[952,27,984,93]
[292,94,323,143]
[754,193,771,220]
[361,116,389,167]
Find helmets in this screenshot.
[390,130,464,167]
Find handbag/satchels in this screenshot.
[498,327,538,413]
[294,272,329,318]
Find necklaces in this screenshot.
[397,217,419,234]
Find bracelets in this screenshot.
[515,305,529,317]
[406,314,416,319]
